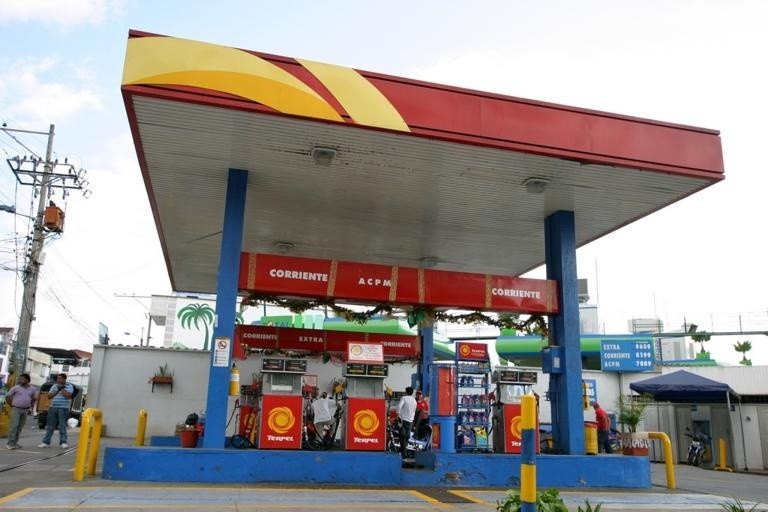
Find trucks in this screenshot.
[37,382,84,428]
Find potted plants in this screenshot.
[692,331,711,359]
[733,341,752,365]
[616,392,656,457]
[152,362,172,384]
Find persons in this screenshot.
[413,389,428,418]
[4,373,37,450]
[592,401,614,453]
[37,373,74,448]
[397,386,417,460]
[307,391,339,442]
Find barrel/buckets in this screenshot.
[181,429,200,448]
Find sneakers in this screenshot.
[59,442,68,449]
[38,444,52,448]
[5,443,21,450]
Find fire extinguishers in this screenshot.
[229,363,240,397]
[582,380,590,411]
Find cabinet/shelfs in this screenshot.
[455,343,492,453]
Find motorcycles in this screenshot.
[684,427,712,466]
[386,424,434,459]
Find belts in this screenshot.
[11,405,31,409]
